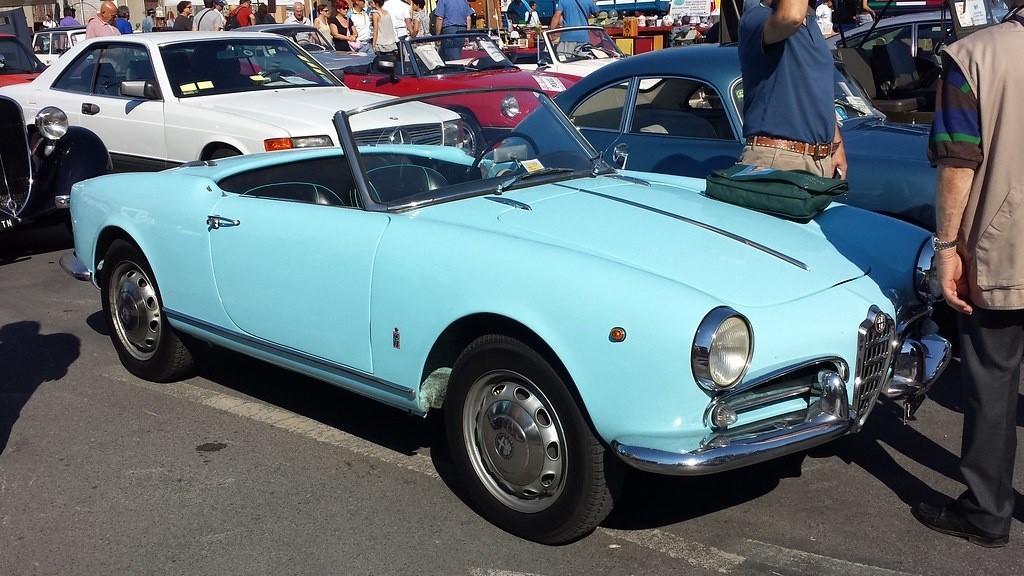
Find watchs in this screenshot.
[931,232,959,251]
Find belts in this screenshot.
[745,136,841,156]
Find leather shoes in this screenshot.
[914,494,1010,547]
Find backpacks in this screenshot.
[225,7,243,31]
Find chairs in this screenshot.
[81,61,118,97]
[162,52,194,94]
[243,182,346,206]
[709,116,736,140]
[349,164,449,208]
[673,30,696,46]
[638,116,718,140]
[439,42,462,62]
[872,44,938,108]
[373,55,406,74]
[125,60,154,81]
[837,48,926,111]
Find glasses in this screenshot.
[412,2,415,6]
[343,6,349,10]
[483,21,487,24]
[186,6,191,9]
[364,1,365,3]
[111,14,117,18]
[323,10,328,12]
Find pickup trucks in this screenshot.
[30,30,87,66]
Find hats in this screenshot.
[214,0,227,7]
[597,12,607,18]
[147,8,156,13]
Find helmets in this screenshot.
[609,9,618,17]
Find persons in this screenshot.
[32,0,875,63]
[912,0,1024,548]
[738,0,848,182]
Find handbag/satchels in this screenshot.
[856,0,873,27]
[705,164,849,224]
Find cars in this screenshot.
[0,33,48,89]
[0,95,113,259]
[483,44,938,233]
[341,33,584,144]
[513,25,628,78]
[824,8,958,52]
[0,30,465,204]
[230,23,376,84]
[59,83,953,547]
